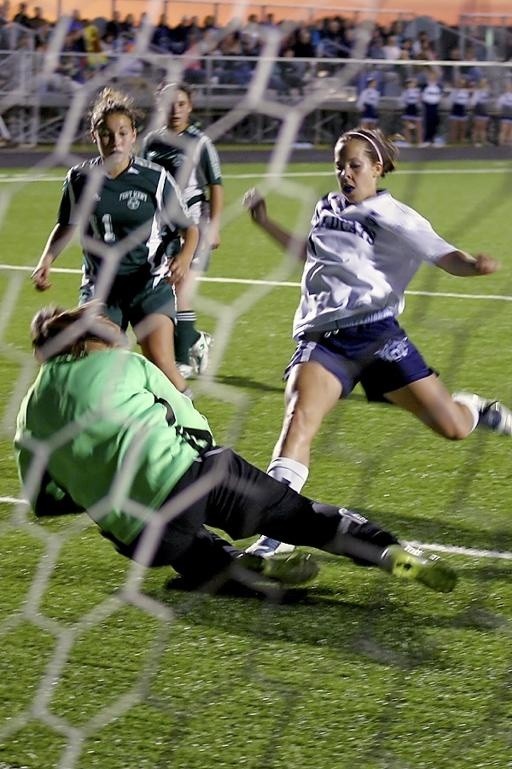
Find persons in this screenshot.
[142,82,224,379]
[0,1,512,148]
[242,124,511,552]
[32,88,194,408]
[15,305,458,603]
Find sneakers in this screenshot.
[175,330,213,379]
[244,535,320,586]
[451,391,512,436]
[380,545,458,594]
